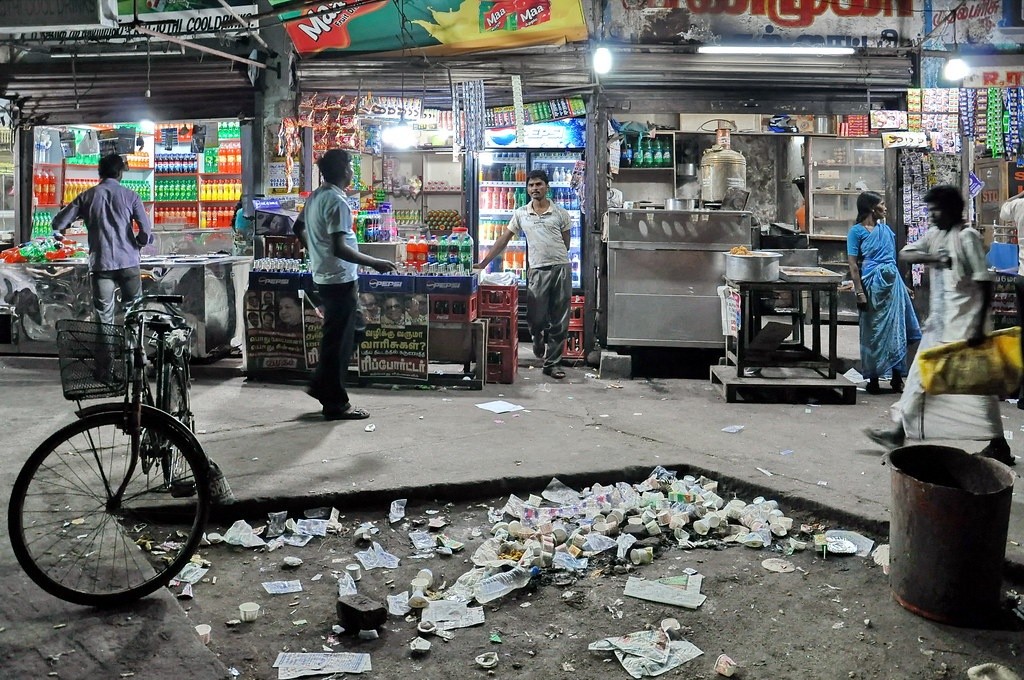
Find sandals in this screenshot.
[532,334,545,358]
[542,366,566,378]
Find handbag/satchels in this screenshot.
[918,326,1022,395]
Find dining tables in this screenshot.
[721,271,842,379]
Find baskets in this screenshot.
[55,318,130,401]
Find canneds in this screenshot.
[840,122,848,137]
[492,153,519,159]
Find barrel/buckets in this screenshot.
[815,113,832,134]
[664,198,694,209]
[986,242,1019,275]
[881,444,1021,625]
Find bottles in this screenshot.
[483,292,510,373]
[33,129,57,204]
[251,238,312,274]
[60,121,242,206]
[132,206,235,231]
[567,295,581,352]
[424,179,461,191]
[473,566,539,604]
[435,300,467,316]
[621,135,673,168]
[477,150,585,290]
[0,211,90,264]
[350,196,474,277]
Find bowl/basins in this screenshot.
[722,249,782,283]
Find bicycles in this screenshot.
[8,294,215,608]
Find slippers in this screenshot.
[326,405,370,421]
[306,386,319,400]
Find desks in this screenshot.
[0,255,254,362]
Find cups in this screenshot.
[160,465,796,677]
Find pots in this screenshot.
[762,234,807,248]
[755,289,792,314]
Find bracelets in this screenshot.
[857,292,864,296]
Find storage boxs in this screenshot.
[249,271,586,384]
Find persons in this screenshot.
[276,292,302,332]
[847,191,923,393]
[246,291,275,331]
[864,185,1016,466]
[231,195,250,234]
[360,293,427,329]
[292,148,398,420]
[51,154,153,380]
[1000,190,1024,410]
[473,169,572,379]
[259,214,293,236]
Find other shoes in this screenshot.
[973,444,1015,464]
[1017,399,1024,408]
[890,380,905,393]
[865,383,880,395]
[862,426,905,450]
